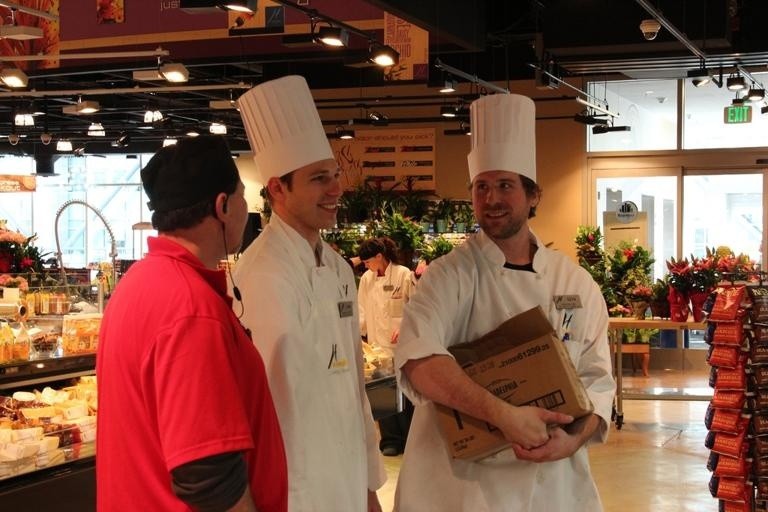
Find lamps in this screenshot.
[727,64,768,114]
[577,97,630,134]
[433,63,510,136]
[0,1,237,154]
[691,79,711,88]
[217,0,400,69]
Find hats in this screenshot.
[138,133,237,211]
[468,92,539,182]
[237,73,336,187]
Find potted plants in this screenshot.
[323,187,472,260]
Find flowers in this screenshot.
[574,223,759,320]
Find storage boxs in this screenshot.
[434,306,595,463]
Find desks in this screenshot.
[0,270,405,512]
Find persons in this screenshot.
[389,170,616,511]
[330,242,364,269]
[228,157,386,512]
[354,235,413,355]
[91,134,288,511]
